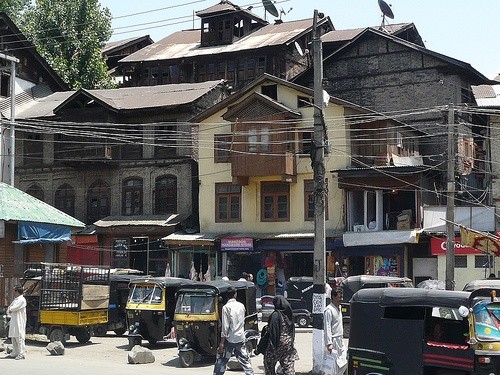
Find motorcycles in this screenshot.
[172,280,259,368]
[284,273,447,339]
[123,276,200,350]
[462,278,500,292]
[0,261,154,346]
[347,286,500,375]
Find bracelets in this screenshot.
[220,343,223,346]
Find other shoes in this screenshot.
[6,355,14,359]
[15,355,25,360]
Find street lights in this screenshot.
[312,89,331,375]
[133,236,150,276]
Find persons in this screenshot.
[5,285,27,360]
[324,286,343,375]
[253,295,295,375]
[238,272,253,282]
[213,286,254,375]
